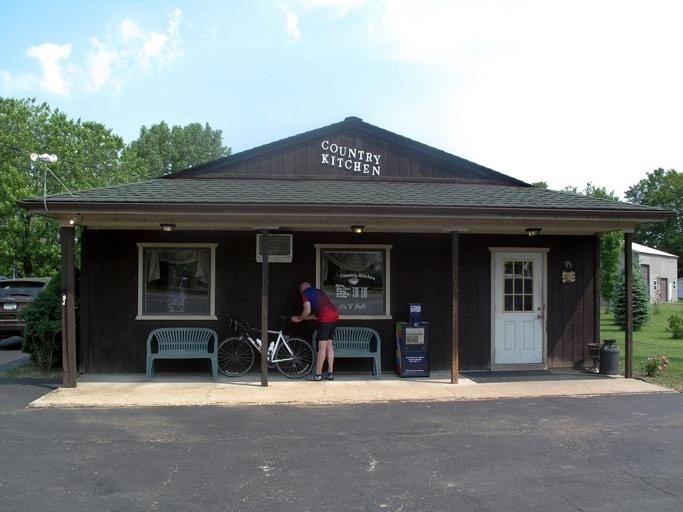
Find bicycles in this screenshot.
[218,315,315,378]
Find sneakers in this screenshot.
[305,373,334,381]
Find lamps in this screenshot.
[351,226,364,233]
[160,224,176,231]
[525,228,542,235]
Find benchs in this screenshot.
[146,327,219,377]
[311,327,381,377]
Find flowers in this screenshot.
[643,355,672,375]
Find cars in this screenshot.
[0,276,52,339]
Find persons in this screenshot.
[291,282,339,380]
